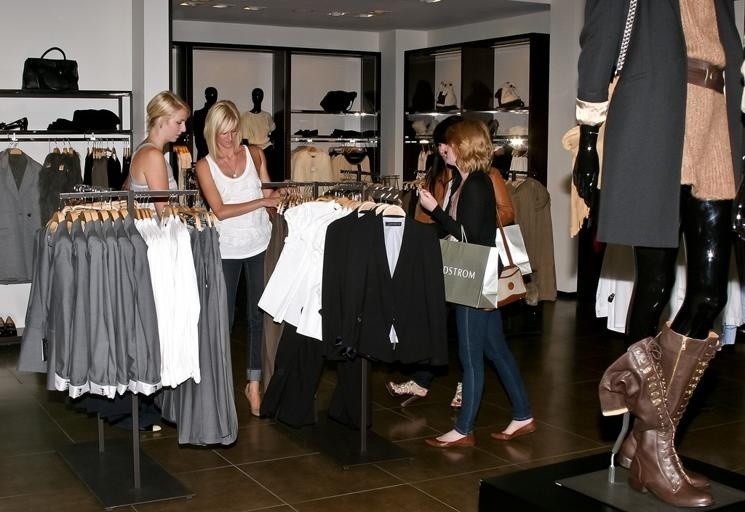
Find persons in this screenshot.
[196,99,302,416]
[385,115,515,418]
[242,88,277,184]
[419,118,537,449]
[193,86,218,159]
[571,0,745,508]
[127,90,192,226]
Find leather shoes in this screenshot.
[490,418,536,441]
[425,429,475,448]
[0,316,17,336]
[140,424,161,432]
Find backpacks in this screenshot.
[321,90,357,115]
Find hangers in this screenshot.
[498,167,533,187]
[5,136,130,171]
[295,137,366,154]
[280,171,427,217]
[488,138,531,158]
[407,137,436,156]
[168,138,192,158]
[46,185,210,234]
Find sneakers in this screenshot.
[295,129,319,138]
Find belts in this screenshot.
[689,57,724,93]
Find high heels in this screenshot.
[451,382,463,412]
[0,117,27,132]
[489,119,499,135]
[384,379,428,406]
[244,383,262,417]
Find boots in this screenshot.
[598,322,723,508]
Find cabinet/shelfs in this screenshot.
[402,33,549,185]
[0,88,134,143]
[170,40,382,181]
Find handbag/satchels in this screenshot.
[439,224,533,312]
[495,81,523,108]
[563,73,620,239]
[418,143,433,172]
[22,47,79,92]
[435,80,458,112]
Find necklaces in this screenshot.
[222,152,239,178]
